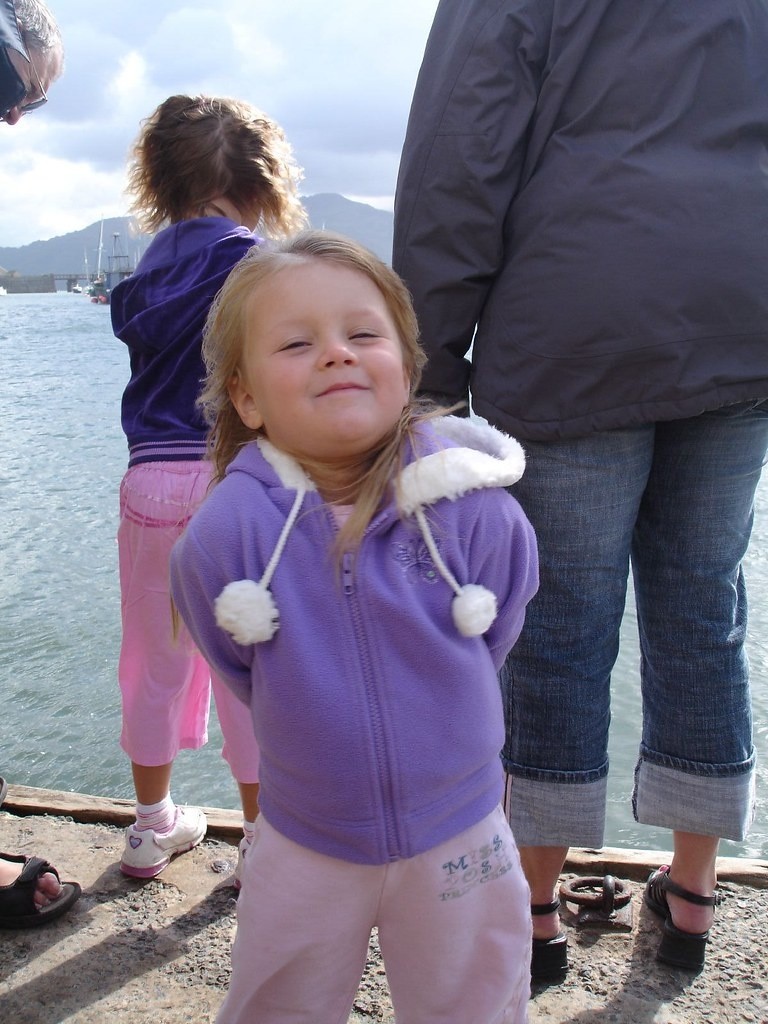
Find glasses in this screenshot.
[0,31,48,122]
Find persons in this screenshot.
[389,0,768,984]
[168,230,538,1023]
[107,94,308,880]
[0,0,81,926]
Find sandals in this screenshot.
[0,852,82,929]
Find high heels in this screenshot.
[645,867,721,971]
[531,891,569,978]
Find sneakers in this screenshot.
[233,837,250,891]
[119,804,207,878]
